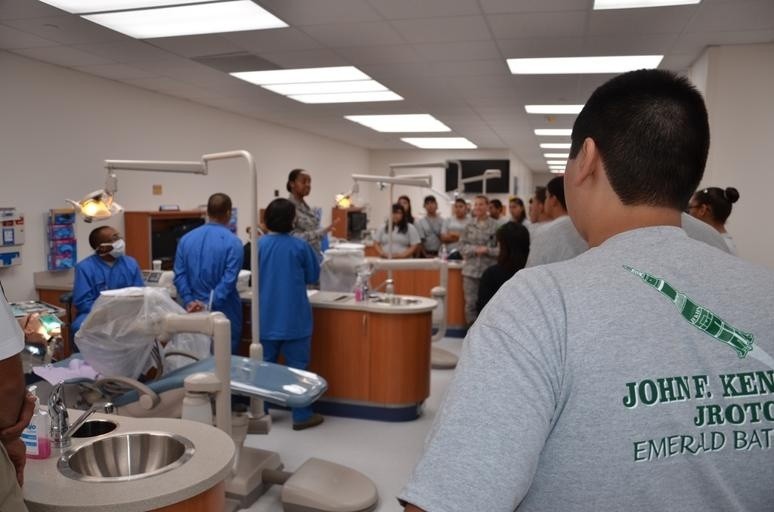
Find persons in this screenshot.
[394,68,774,512]
[69,225,146,354]
[679,186,740,257]
[0,273,37,512]
[366,169,590,325]
[248,197,324,431]
[284,168,341,291]
[173,193,245,357]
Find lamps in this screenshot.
[335,193,351,209]
[64,189,124,224]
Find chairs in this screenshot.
[33,352,329,409]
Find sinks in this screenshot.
[71,418,120,439]
[56,430,195,483]
[370,296,423,306]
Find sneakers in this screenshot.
[292,412,323,429]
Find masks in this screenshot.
[109,239,126,258]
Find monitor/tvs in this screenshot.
[445,160,509,193]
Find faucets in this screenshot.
[46,379,114,447]
[369,280,394,298]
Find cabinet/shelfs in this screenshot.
[367,256,467,337]
[122,210,207,269]
[236,287,438,422]
